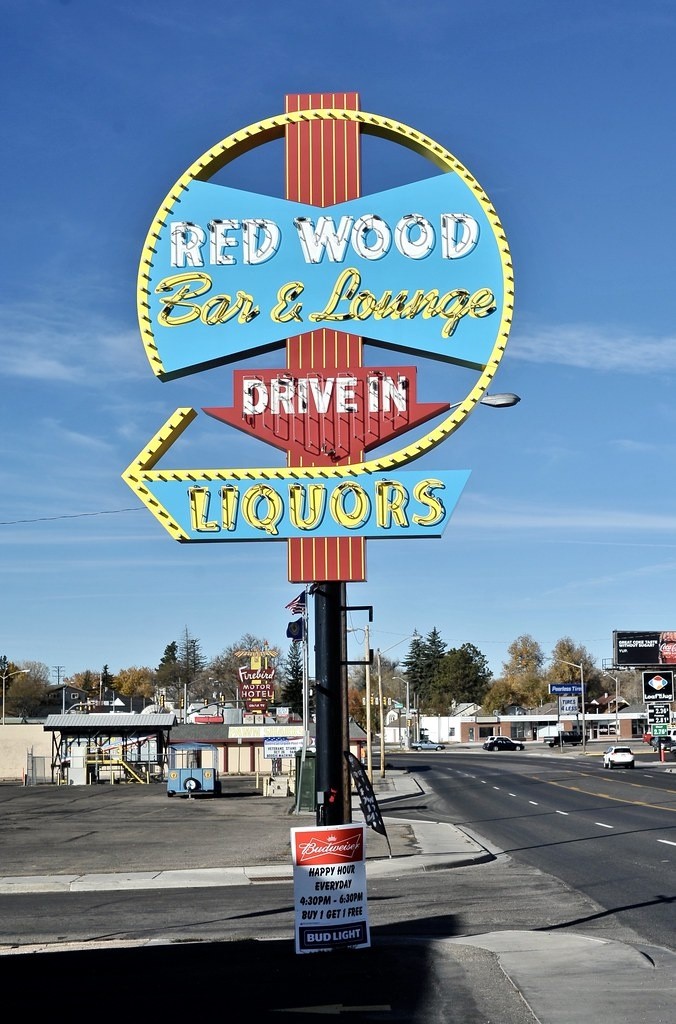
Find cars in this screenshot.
[482,739,525,751]
[642,728,676,746]
[411,739,445,751]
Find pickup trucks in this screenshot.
[543,730,589,747]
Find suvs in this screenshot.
[603,745,635,770]
[485,736,521,743]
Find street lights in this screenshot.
[183,677,214,724]
[0,668,30,725]
[376,635,423,780]
[602,666,618,743]
[347,625,374,791]
[392,676,410,750]
[544,657,587,753]
